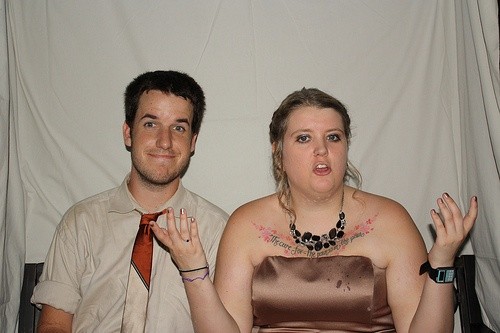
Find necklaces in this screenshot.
[286,185,346,251]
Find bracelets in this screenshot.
[178,266,209,282]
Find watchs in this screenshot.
[419,261,456,283]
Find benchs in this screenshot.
[455,255,499,333]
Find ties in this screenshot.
[120,208,167,333]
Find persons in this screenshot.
[31,70,231,333]
[148,87,479,333]
